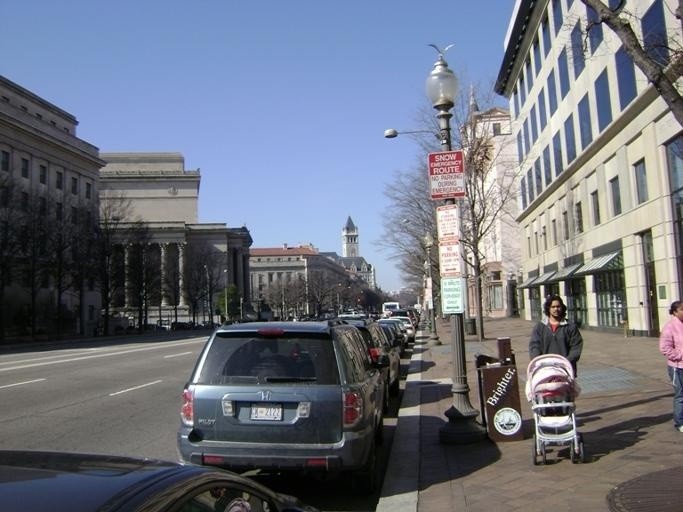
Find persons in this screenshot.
[528,295,582,378]
[659,300,682,433]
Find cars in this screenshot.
[178,301,419,493]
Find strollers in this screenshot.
[524,353,584,466]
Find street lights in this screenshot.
[421,231,441,347]
[383,40,489,448]
[223,269,228,321]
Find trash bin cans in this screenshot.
[465,319,476,335]
[477,338,524,442]
